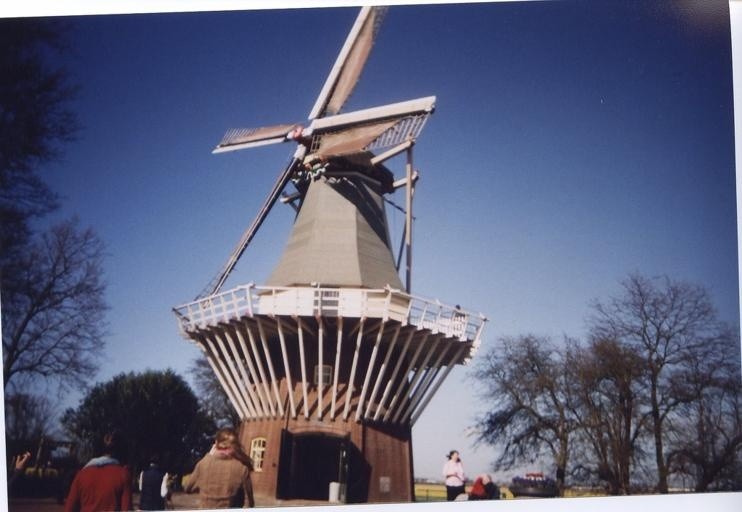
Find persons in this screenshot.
[137,456,169,511]
[62,429,134,512]
[6,450,31,487]
[183,427,256,509]
[441,450,515,501]
